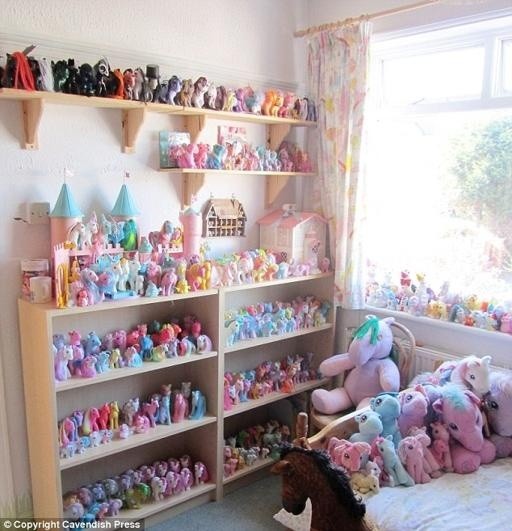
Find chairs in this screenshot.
[307,321,415,439]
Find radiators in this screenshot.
[342,327,512,392]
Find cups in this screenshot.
[30,276,52,304]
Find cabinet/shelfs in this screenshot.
[0,85,317,207]
[16,270,338,531]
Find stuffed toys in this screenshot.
[360,268,512,336]
[0,44,336,531]
[311,313,402,417]
[270,436,379,531]
[327,353,511,495]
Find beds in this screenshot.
[292,404,511,531]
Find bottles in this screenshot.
[21,259,49,303]
